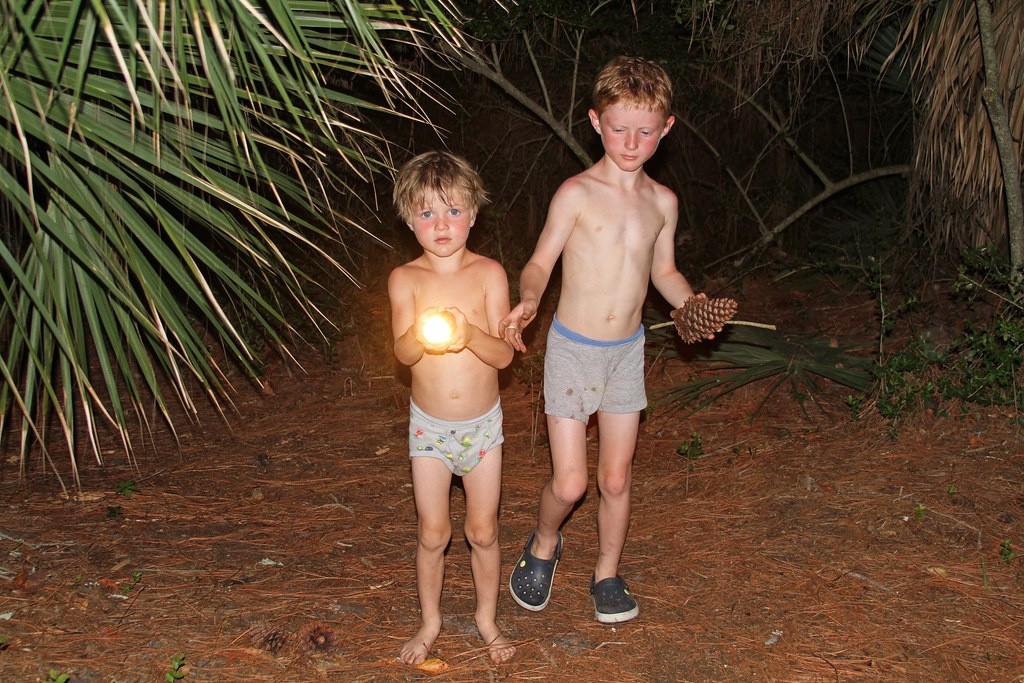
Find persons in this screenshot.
[497,54,721,623]
[388,151,518,665]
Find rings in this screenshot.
[506,326,517,330]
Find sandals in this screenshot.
[509,527,563,611]
[590,570,639,622]
